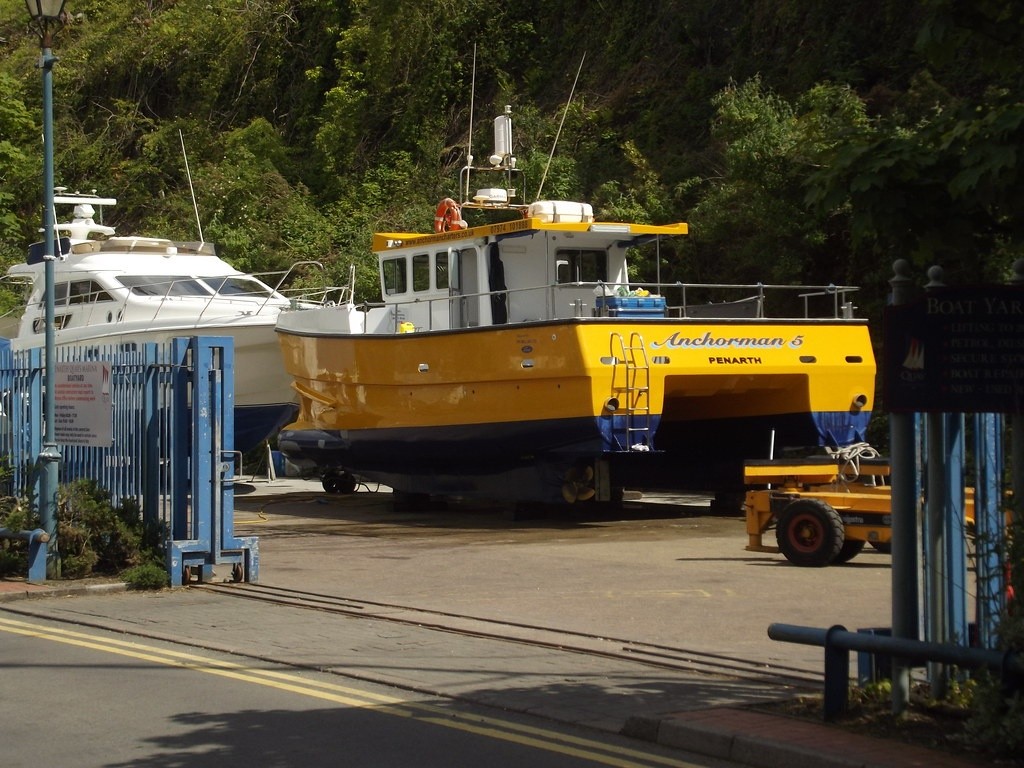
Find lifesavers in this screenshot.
[435,198,461,233]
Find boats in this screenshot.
[0,127,323,492]
[274,44,878,465]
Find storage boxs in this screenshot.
[596,297,666,317]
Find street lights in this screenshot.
[24,1,76,580]
